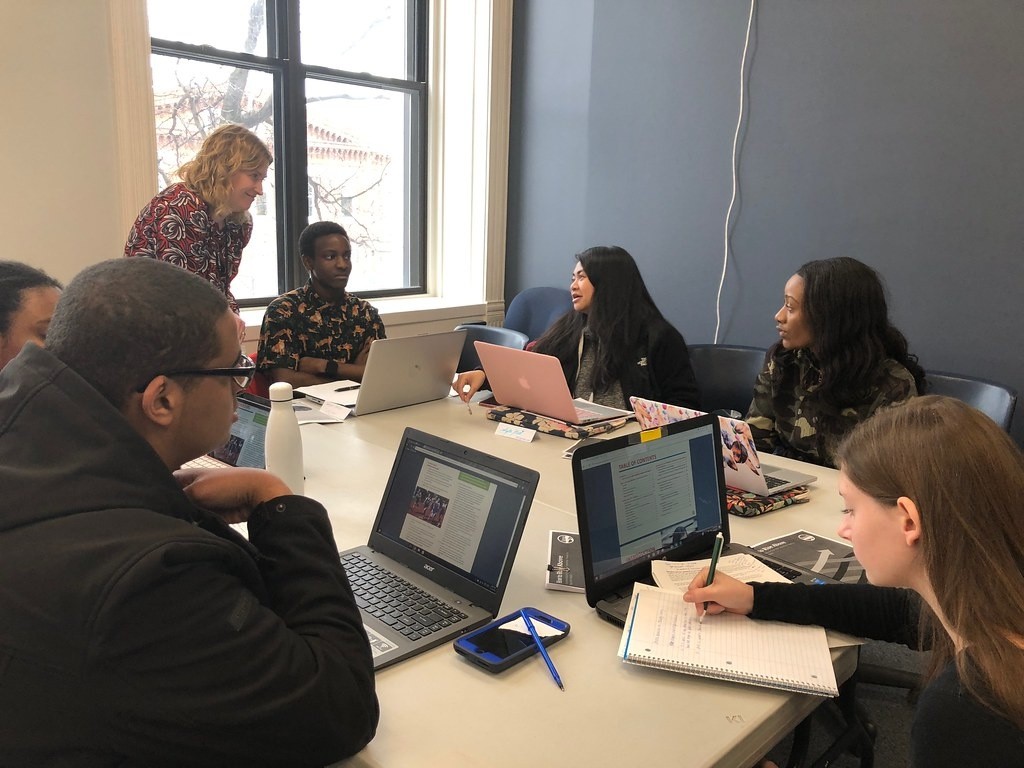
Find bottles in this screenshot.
[265,380,305,496]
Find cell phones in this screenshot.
[453,607,570,675]
[478,396,502,407]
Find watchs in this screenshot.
[325,360,338,377]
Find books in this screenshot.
[618,582,840,697]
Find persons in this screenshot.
[0,257,380,768]
[452,245,697,411]
[223,437,242,460]
[411,488,447,527]
[682,395,1024,768]
[124,125,274,345]
[741,257,926,471]
[255,221,387,398]
[0,262,63,371]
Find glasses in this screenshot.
[136,354,257,395]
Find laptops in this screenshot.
[572,412,846,629]
[338,427,539,673]
[473,340,635,424]
[306,329,469,416]
[179,391,273,473]
[628,396,817,497]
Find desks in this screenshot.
[230,378,859,768]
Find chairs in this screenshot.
[827,370,1017,758]
[502,287,575,343]
[454,325,529,373]
[687,344,768,419]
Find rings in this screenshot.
[242,332,246,335]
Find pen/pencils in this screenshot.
[520,608,565,692]
[335,385,360,392]
[699,532,724,624]
[464,393,472,415]
